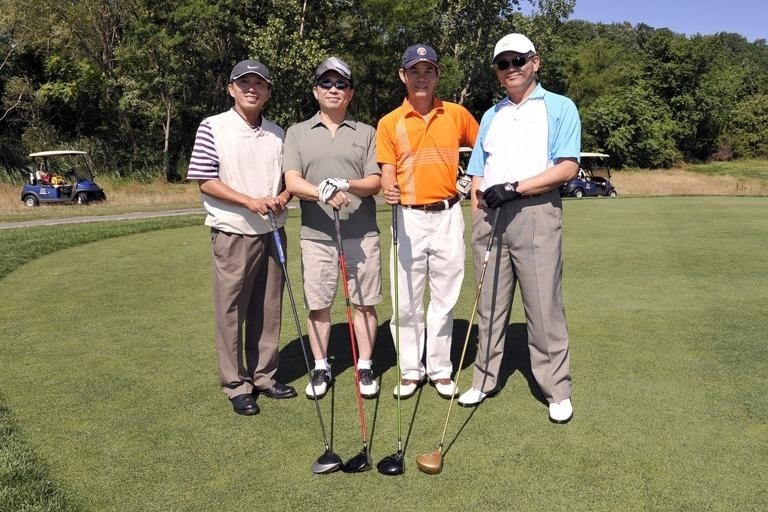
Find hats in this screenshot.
[314,55,354,81]
[491,32,537,65]
[229,59,270,84]
[401,43,439,69]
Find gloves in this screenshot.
[314,177,351,205]
[454,174,473,203]
[482,180,523,211]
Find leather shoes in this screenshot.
[229,392,260,416]
[547,398,574,424]
[431,378,459,399]
[256,381,298,399]
[458,386,488,407]
[356,368,381,399]
[304,363,335,400]
[393,378,423,401]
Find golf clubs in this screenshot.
[333,208,372,473]
[416,207,502,473]
[377,204,404,474]
[267,208,342,473]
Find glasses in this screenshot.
[316,79,352,90]
[494,53,535,71]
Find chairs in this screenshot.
[36,170,52,185]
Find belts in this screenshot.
[396,192,461,214]
[512,191,541,201]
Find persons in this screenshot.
[187,61,297,416]
[283,57,383,399]
[458,32,581,424]
[375,44,480,398]
